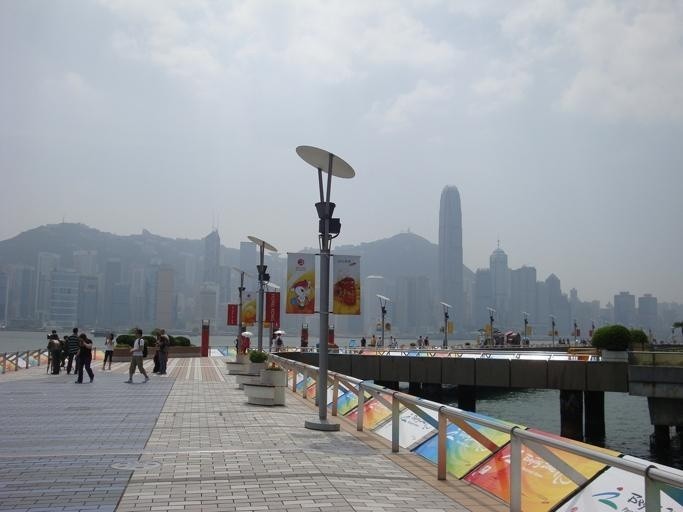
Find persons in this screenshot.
[232,326,593,357]
[44,326,170,385]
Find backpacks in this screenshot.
[277,338,282,345]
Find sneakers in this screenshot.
[75,374,94,384]
[125,378,149,383]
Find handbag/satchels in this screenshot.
[142,342,148,357]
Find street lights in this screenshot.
[292,142,358,433]
[246,234,278,352]
[374,292,596,350]
[231,264,255,363]
